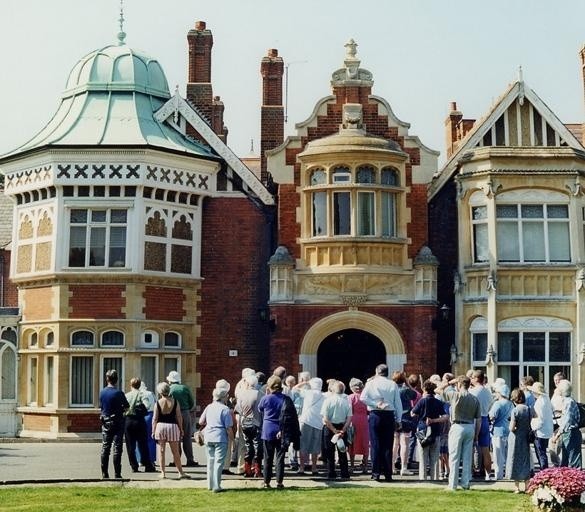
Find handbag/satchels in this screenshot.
[133,402,148,417]
[528,430,535,443]
[415,425,436,448]
[194,430,204,446]
[553,423,559,430]
[242,417,257,437]
[577,402,585,428]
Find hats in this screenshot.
[491,377,510,399]
[556,379,572,395]
[216,379,231,393]
[213,388,227,401]
[166,371,181,382]
[351,378,364,391]
[256,371,266,381]
[268,377,281,389]
[527,382,547,395]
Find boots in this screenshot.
[253,455,263,477]
[244,452,254,477]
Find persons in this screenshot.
[100,366,582,491]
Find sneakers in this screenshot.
[187,462,198,466]
[179,473,190,478]
[400,469,414,475]
[261,483,284,489]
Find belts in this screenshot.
[454,421,469,424]
